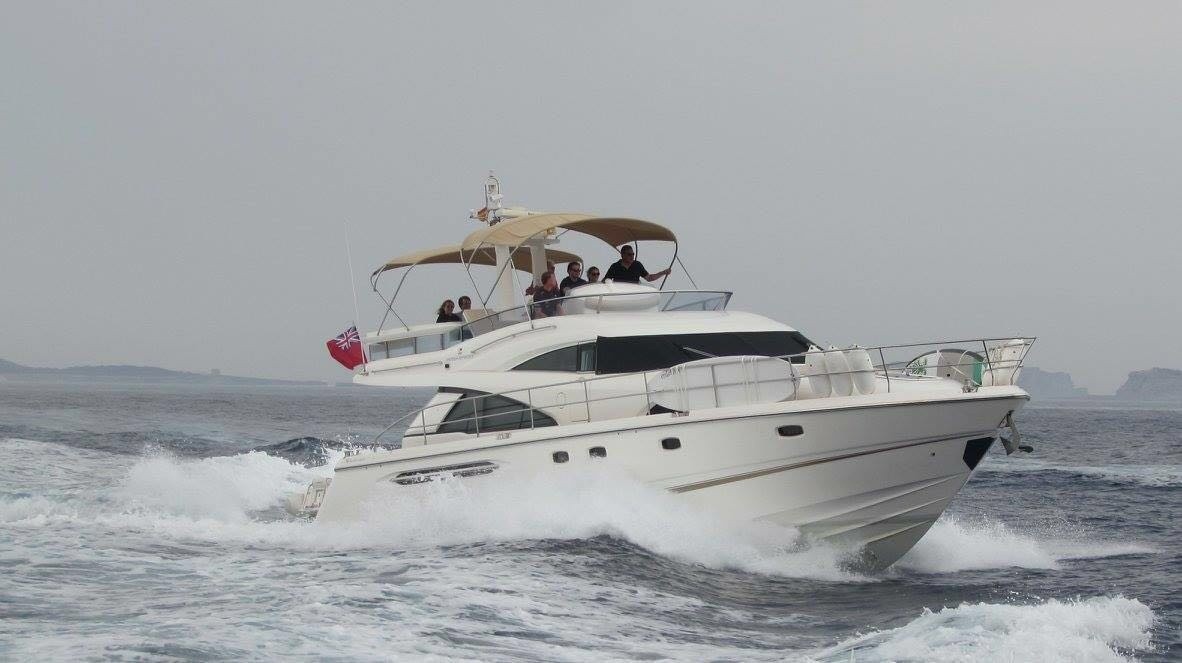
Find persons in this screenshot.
[601,245,671,284]
[526,261,601,320]
[436,296,471,323]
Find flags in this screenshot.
[326,326,368,371]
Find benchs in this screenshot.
[463,307,520,338]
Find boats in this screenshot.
[302,167,1037,573]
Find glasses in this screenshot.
[591,273,600,275]
[625,253,634,256]
[571,269,581,272]
[548,267,555,269]
[446,304,454,309]
